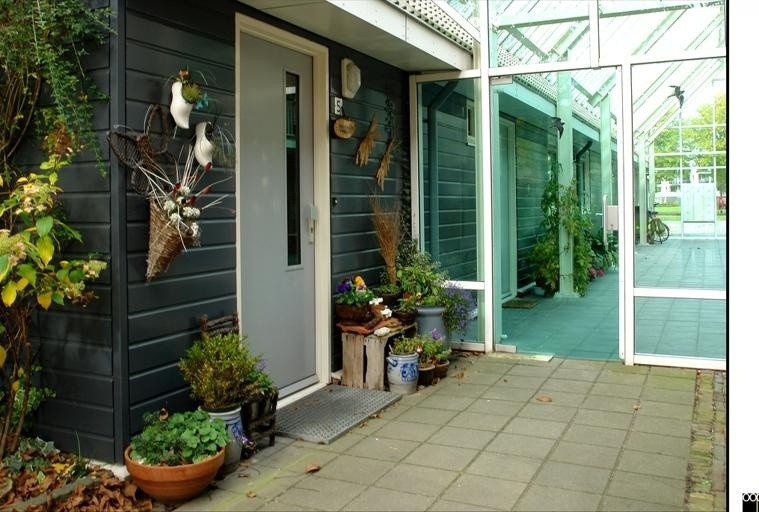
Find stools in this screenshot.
[338,322,418,389]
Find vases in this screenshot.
[334,301,370,326]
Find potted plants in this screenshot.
[123,328,274,506]
[378,233,479,395]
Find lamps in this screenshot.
[340,58,362,99]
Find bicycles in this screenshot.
[648,204,670,244]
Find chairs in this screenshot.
[201,311,279,459]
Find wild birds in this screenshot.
[668,86,685,109]
[549,117,565,139]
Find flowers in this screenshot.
[334,275,392,319]
